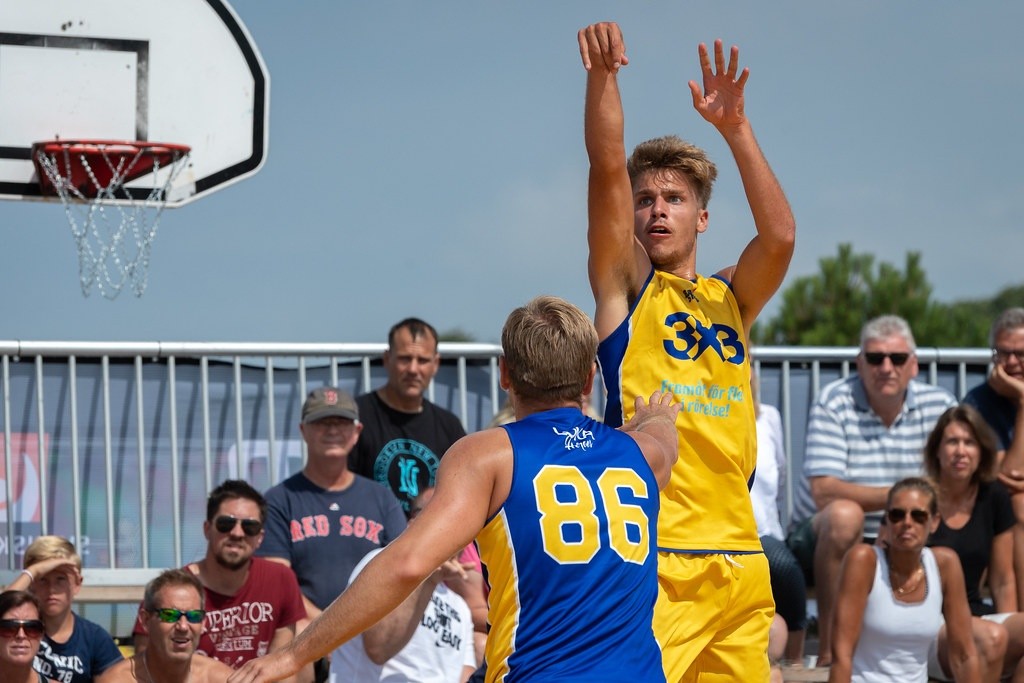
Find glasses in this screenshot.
[992,347,1024,364]
[216,515,264,537]
[146,608,205,623]
[887,509,931,524]
[311,420,353,431]
[865,353,913,366]
[0,619,45,638]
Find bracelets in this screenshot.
[21,569,34,584]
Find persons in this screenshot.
[576,20,809,683]
[253,387,407,683]
[803,315,958,667]
[348,318,468,522]
[926,404,1024,683]
[960,307,1024,612]
[227,296,683,683]
[0,535,125,683]
[133,480,308,683]
[99,569,237,683]
[0,590,61,683]
[828,477,982,683]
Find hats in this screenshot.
[302,387,359,422]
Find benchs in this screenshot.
[73,566,177,604]
